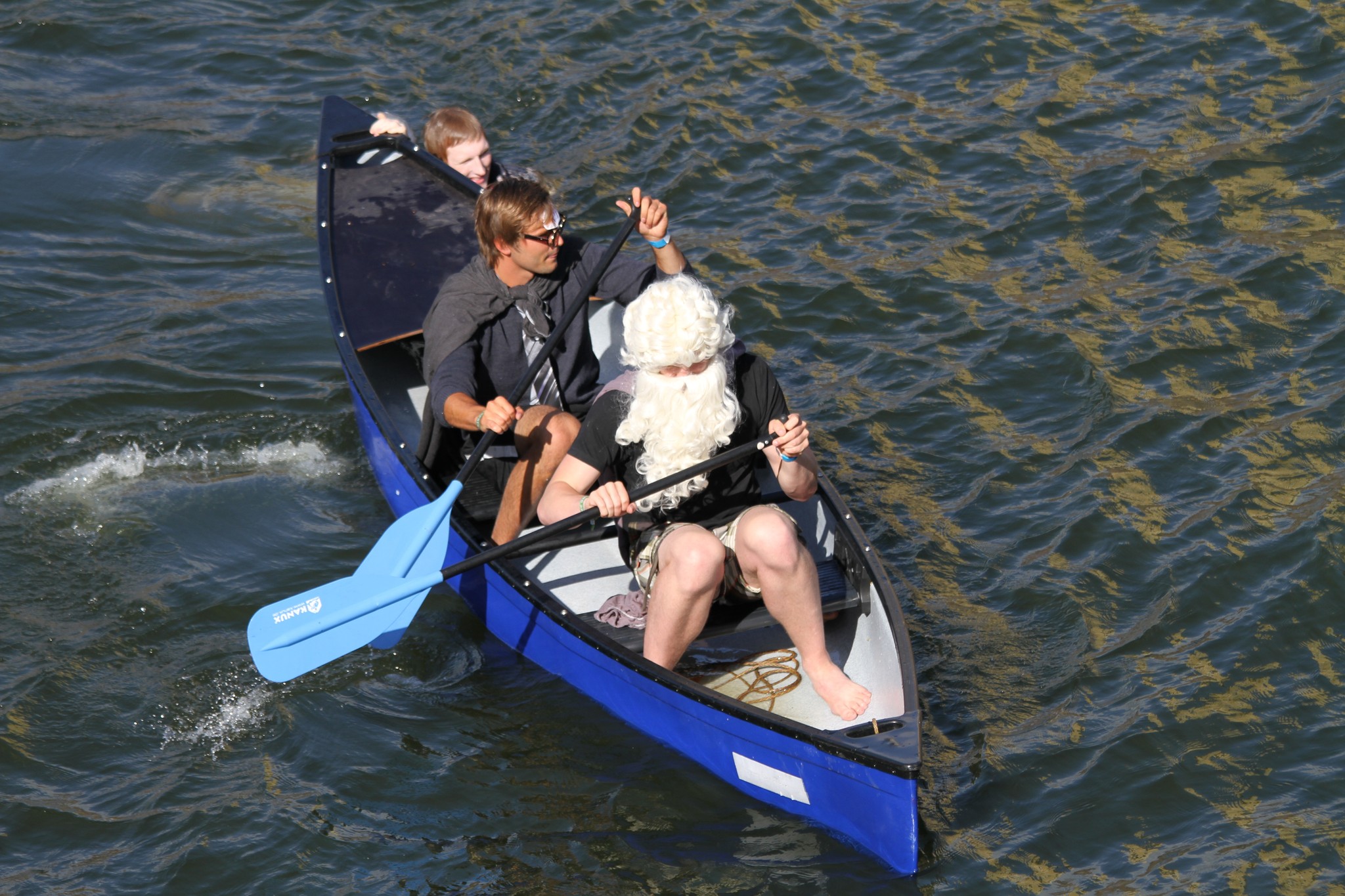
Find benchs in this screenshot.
[441,468,503,526]
[575,557,861,654]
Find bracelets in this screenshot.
[776,447,797,462]
[476,410,485,430]
[643,229,670,248]
[580,496,600,531]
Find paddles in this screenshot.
[247,415,801,682]
[355,203,643,652]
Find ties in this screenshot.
[516,308,564,413]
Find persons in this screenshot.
[369,107,511,189]
[417,175,693,545]
[536,274,872,720]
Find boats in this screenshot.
[314,92,923,879]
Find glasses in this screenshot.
[512,212,566,250]
